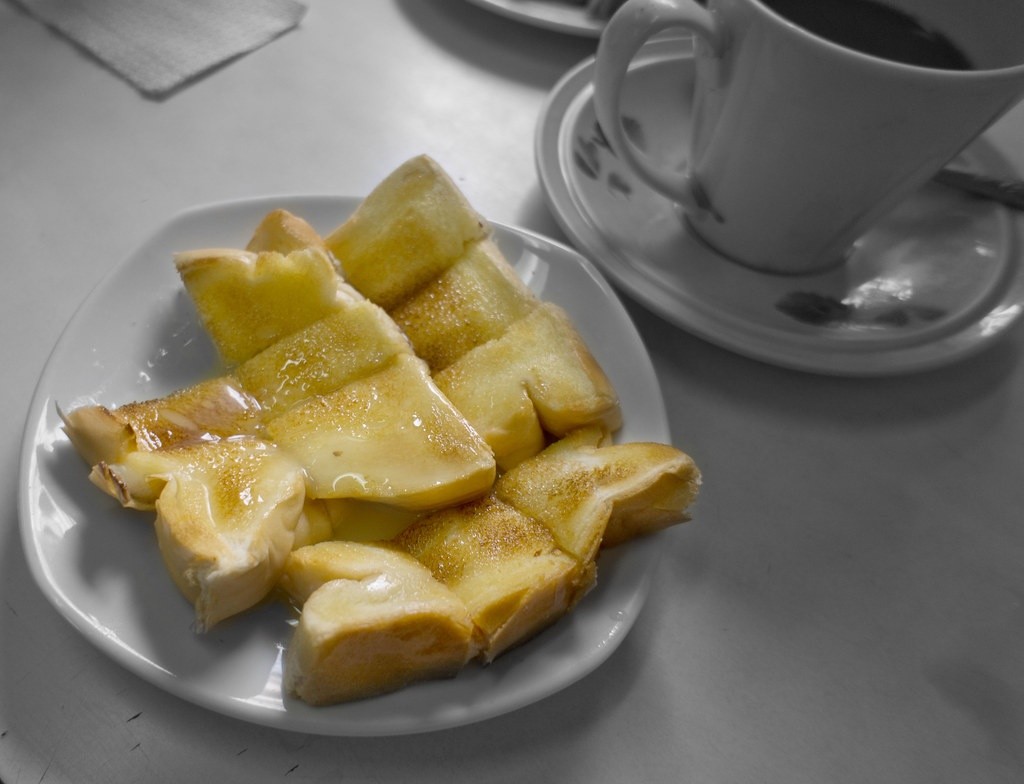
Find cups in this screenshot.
[593,0,1024,273]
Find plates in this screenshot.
[18,198,669,736]
[472,0,605,35]
[534,37,1024,374]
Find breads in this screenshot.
[53,154,700,708]
